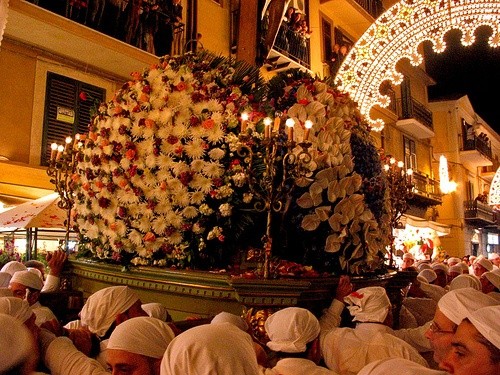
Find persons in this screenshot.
[0,247,500,375]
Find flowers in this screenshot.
[69,49,391,276]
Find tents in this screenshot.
[0,192,76,260]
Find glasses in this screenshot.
[429,323,455,335]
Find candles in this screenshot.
[64,136,73,152]
[383,157,413,184]
[286,118,295,141]
[240,112,248,133]
[55,145,64,162]
[50,143,58,161]
[304,120,314,140]
[72,133,81,151]
[273,115,281,133]
[263,116,272,140]
